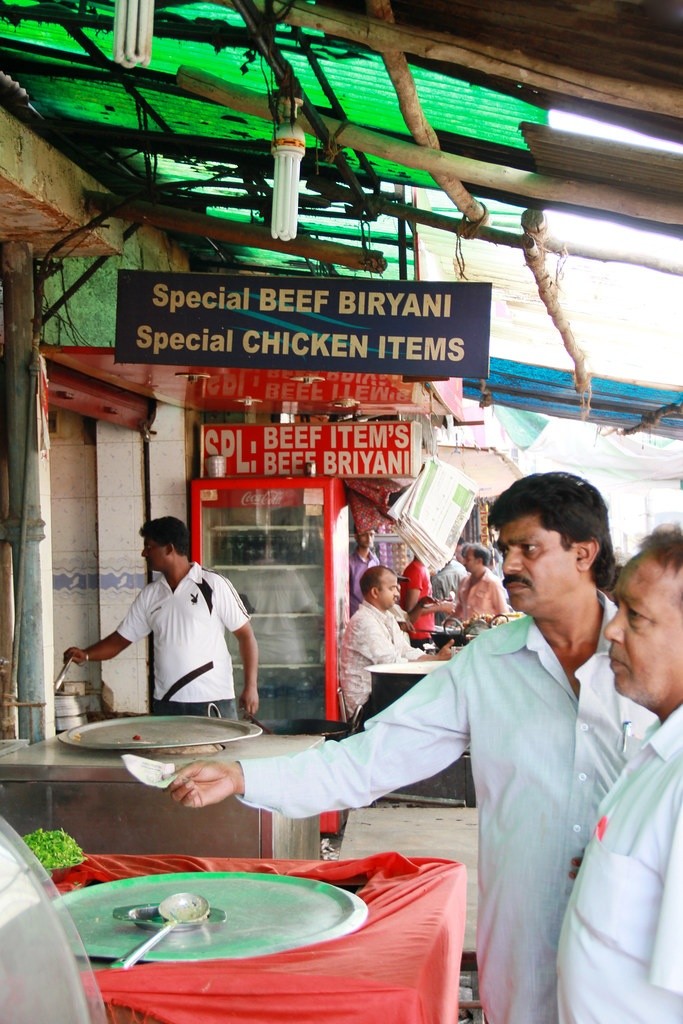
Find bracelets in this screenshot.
[78,649,89,667]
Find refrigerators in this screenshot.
[189,474,350,740]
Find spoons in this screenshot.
[106,893,210,969]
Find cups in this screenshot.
[207,455,227,478]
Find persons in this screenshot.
[557,525,683,1023]
[340,524,509,726]
[63,516,259,720]
[162,473,661,1024]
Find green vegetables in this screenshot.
[22,827,88,869]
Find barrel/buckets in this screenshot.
[363,656,456,717]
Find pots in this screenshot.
[53,691,88,731]
[431,614,509,651]
[257,718,350,742]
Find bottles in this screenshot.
[240,667,325,722]
[305,461,317,478]
[217,528,325,565]
